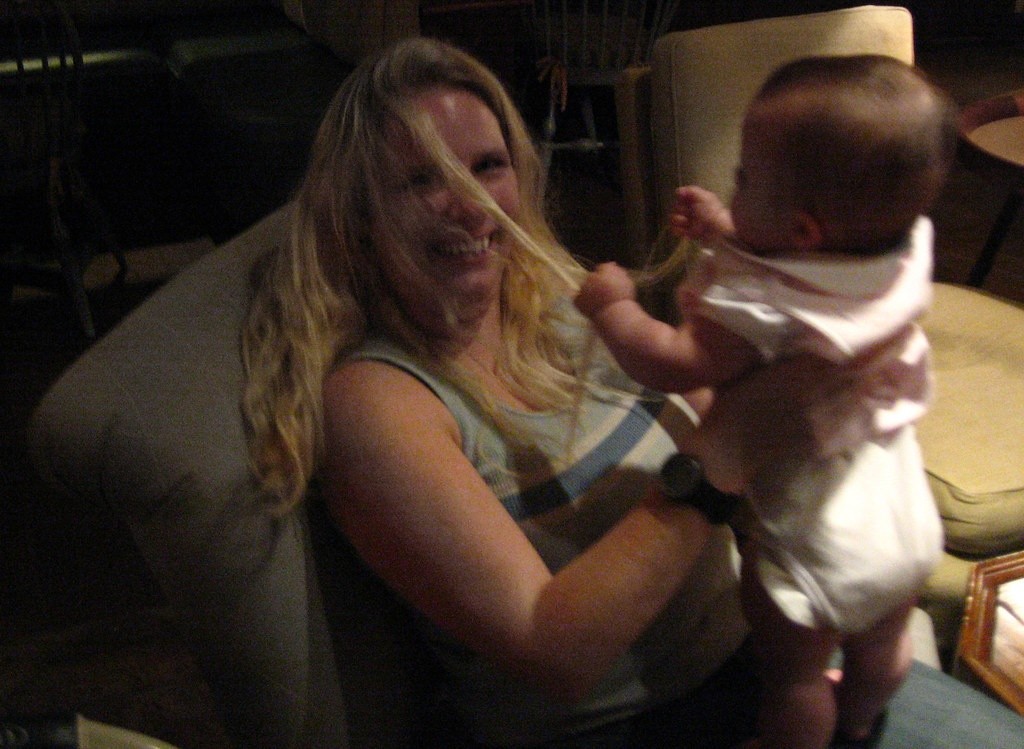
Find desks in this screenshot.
[965,115,1024,289]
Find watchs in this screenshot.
[659,448,742,530]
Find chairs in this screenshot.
[525,0,675,204]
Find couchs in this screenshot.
[19,194,946,749]
[618,2,1024,676]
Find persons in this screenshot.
[573,51,944,749]
[305,31,879,749]
